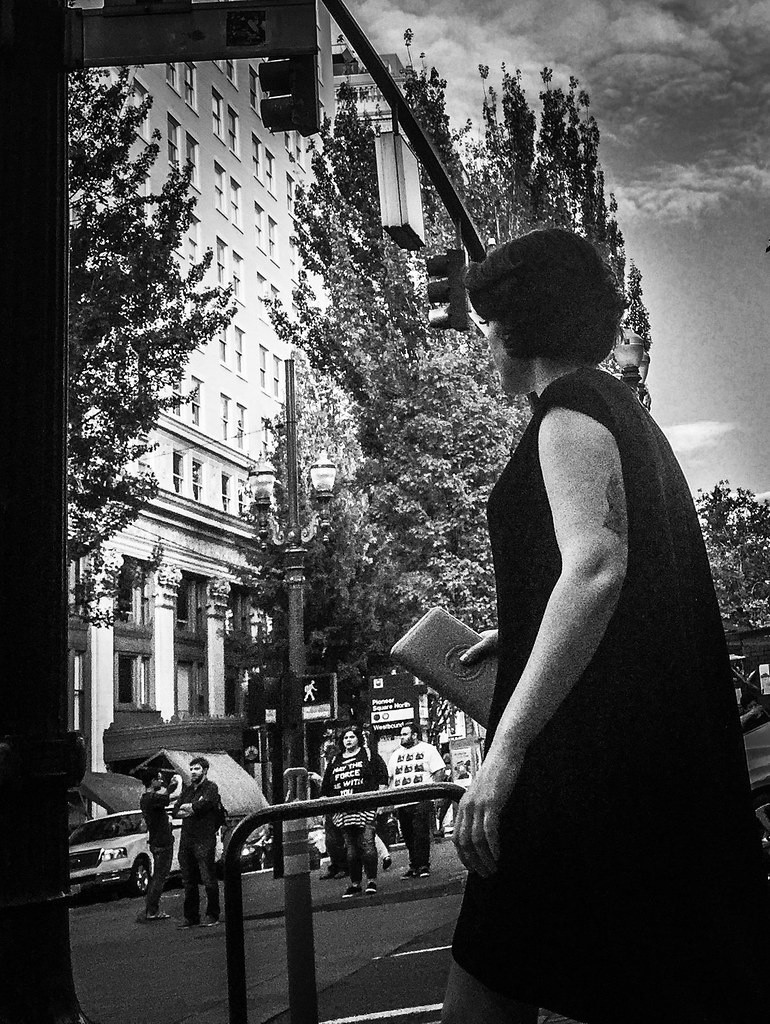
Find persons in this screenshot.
[387,723,455,881]
[739,692,770,733]
[320,724,394,900]
[172,757,221,931]
[137,764,182,922]
[440,225,770,1024]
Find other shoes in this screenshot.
[319,869,346,879]
[382,856,392,871]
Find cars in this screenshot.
[68,807,225,902]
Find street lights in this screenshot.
[235,356,342,832]
[614,325,652,414]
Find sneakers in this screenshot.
[198,916,220,927]
[146,910,171,920]
[400,866,430,880]
[177,919,200,930]
[341,885,363,898]
[365,882,377,894]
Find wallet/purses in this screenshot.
[389,607,494,731]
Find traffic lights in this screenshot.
[260,5,332,137]
[422,249,469,333]
[301,675,330,702]
